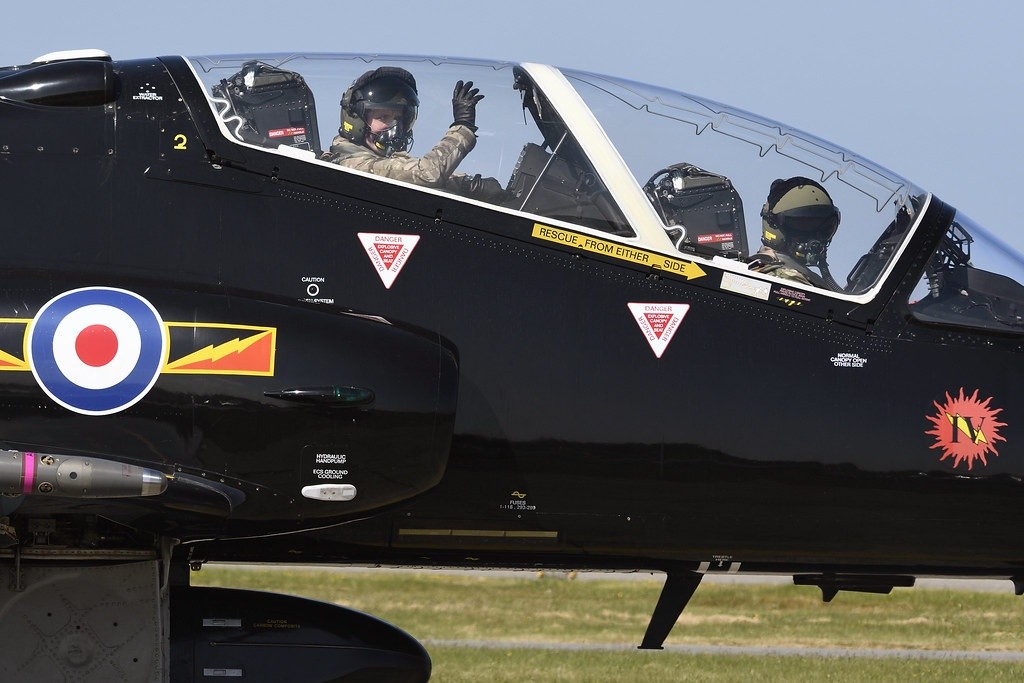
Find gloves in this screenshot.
[462,174,503,202]
[449,80,485,137]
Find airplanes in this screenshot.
[0,55,1024,682]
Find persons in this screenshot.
[330,67,510,205]
[757,176,841,286]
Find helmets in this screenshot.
[339,65,421,146]
[760,177,841,257]
